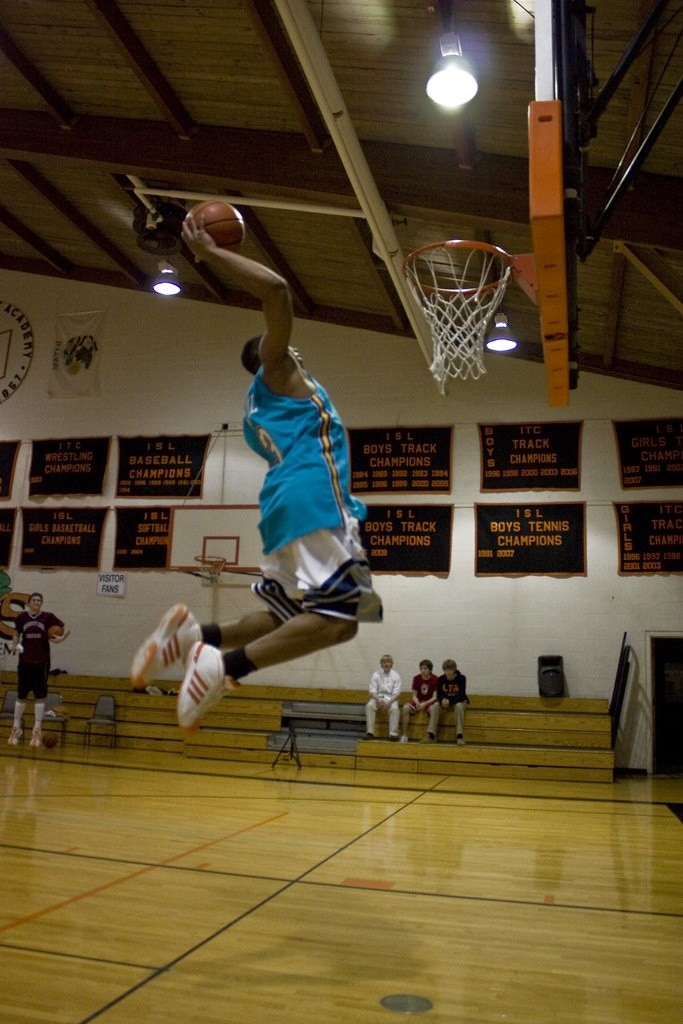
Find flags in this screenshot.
[47,311,106,399]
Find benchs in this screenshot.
[1,668,614,786]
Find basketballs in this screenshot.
[185,199,245,253]
[47,626,64,640]
[42,732,58,748]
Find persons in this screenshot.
[130,214,384,733]
[7,593,70,747]
[399,659,469,745]
[361,655,401,742]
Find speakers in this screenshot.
[537,655,565,697]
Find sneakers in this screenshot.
[29,728,42,746]
[177,642,239,729]
[128,605,199,688]
[7,728,22,745]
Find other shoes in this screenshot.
[362,734,372,740]
[422,737,436,743]
[457,738,464,745]
[400,736,408,742]
[391,736,398,741]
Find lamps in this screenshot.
[152,259,182,296]
[485,308,516,354]
[426,9,480,107]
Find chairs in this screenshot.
[33,689,68,748]
[82,693,118,749]
[0,688,25,746]
